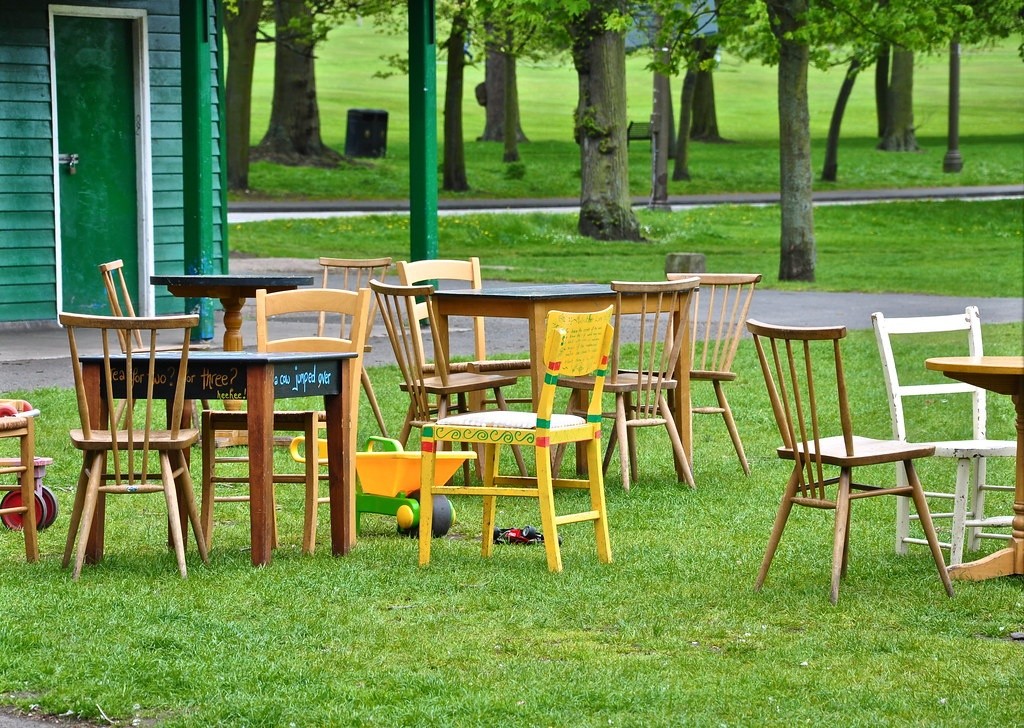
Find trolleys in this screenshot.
[290,434,477,537]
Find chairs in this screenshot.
[0,397,42,565]
[870,305,1012,565]
[55,311,210,577]
[99,259,204,447]
[599,273,763,483]
[368,277,527,485]
[746,318,953,605]
[200,288,375,556]
[396,256,531,482]
[317,256,392,438]
[551,278,700,491]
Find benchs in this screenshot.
[627,120,652,154]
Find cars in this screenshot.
[492,526,562,547]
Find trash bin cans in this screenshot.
[346,109,388,158]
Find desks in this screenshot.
[924,356,1024,581]
[79,351,361,568]
[429,282,698,494]
[150,275,315,448]
